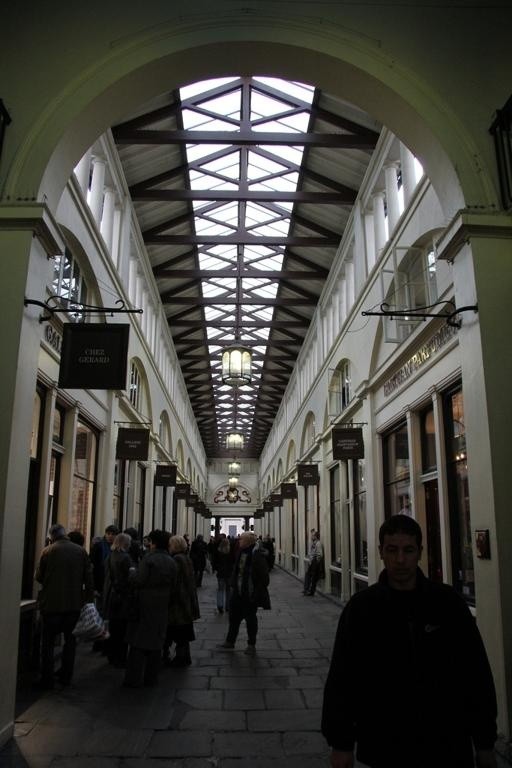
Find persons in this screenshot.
[207,533,274,654]
[320,514,498,768]
[35,524,210,694]
[303,533,323,595]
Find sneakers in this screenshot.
[215,640,235,649]
[245,640,255,648]
[172,656,191,666]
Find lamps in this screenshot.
[220,328,252,386]
[226,429,245,452]
[229,476,239,488]
[227,488,238,503]
[227,461,241,475]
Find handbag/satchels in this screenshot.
[72,603,111,645]
